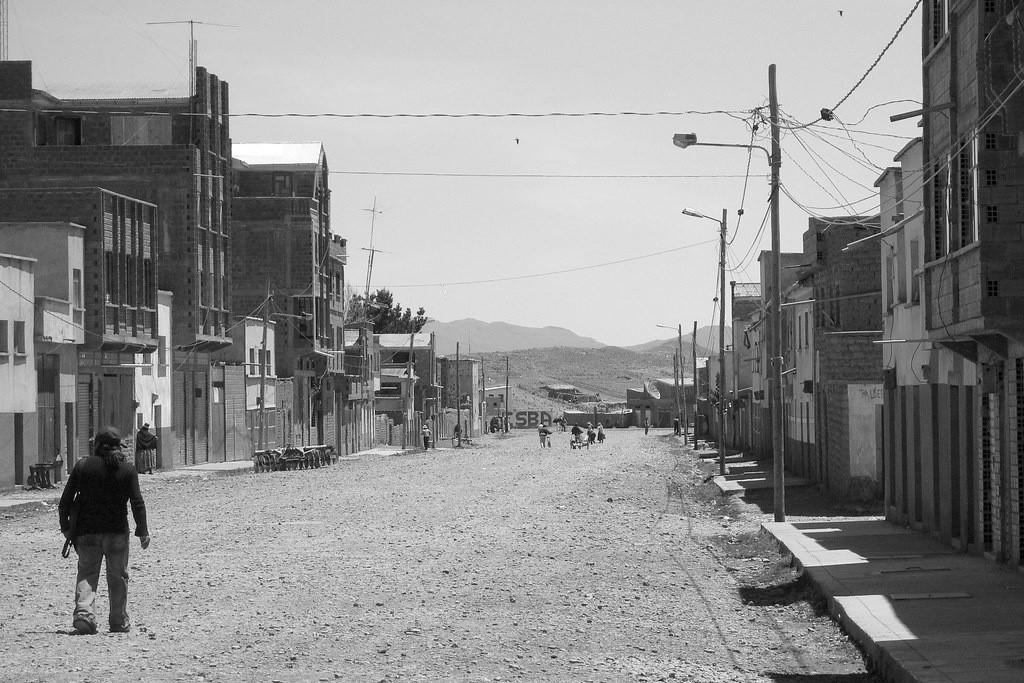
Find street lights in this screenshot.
[258,312,315,450]
[680,205,728,475]
[656,321,688,445]
[671,132,786,522]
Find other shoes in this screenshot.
[110,626,130,632]
[74,614,96,634]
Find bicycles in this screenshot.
[546,433,553,447]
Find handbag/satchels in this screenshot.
[61,457,86,537]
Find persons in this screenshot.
[59,426,150,633]
[538,417,567,448]
[137,423,158,474]
[455,423,463,438]
[421,425,432,450]
[674,417,679,433]
[570,422,606,449]
[644,417,649,435]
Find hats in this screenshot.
[423,425,427,428]
[143,423,150,429]
[89,426,121,446]
